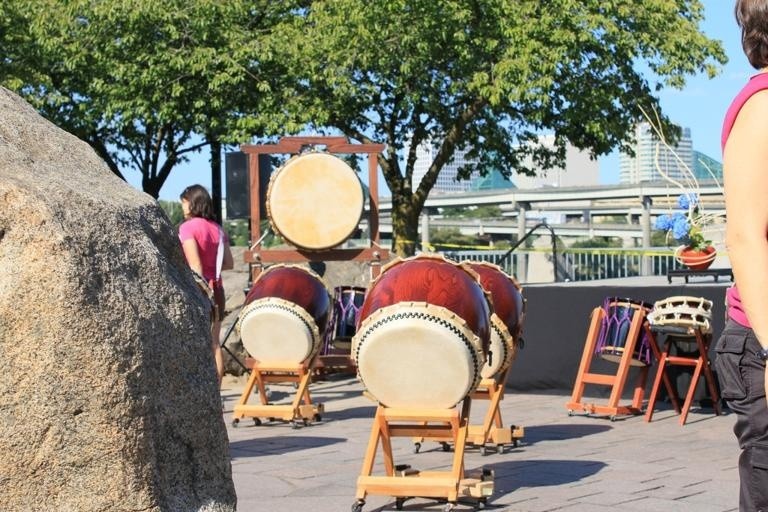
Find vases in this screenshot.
[676,245,715,272]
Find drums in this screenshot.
[237,264,333,365]
[596,296,654,366]
[351,253,492,410]
[647,296,714,337]
[336,286,366,348]
[266,152,365,253]
[460,260,527,379]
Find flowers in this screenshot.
[655,191,713,254]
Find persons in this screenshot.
[713,1,768,512]
[178,185,233,392]
